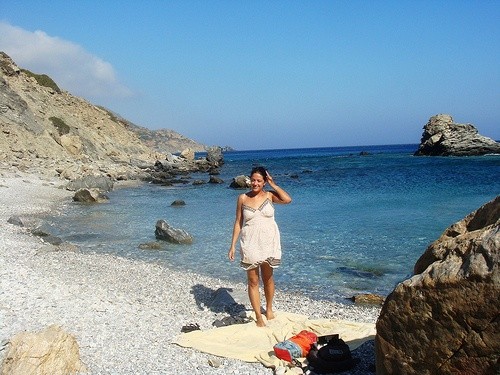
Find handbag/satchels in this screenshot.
[273,329,318,362]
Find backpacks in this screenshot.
[307,334,361,374]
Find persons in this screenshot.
[228,166,292,328]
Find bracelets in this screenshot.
[274,186,278,190]
